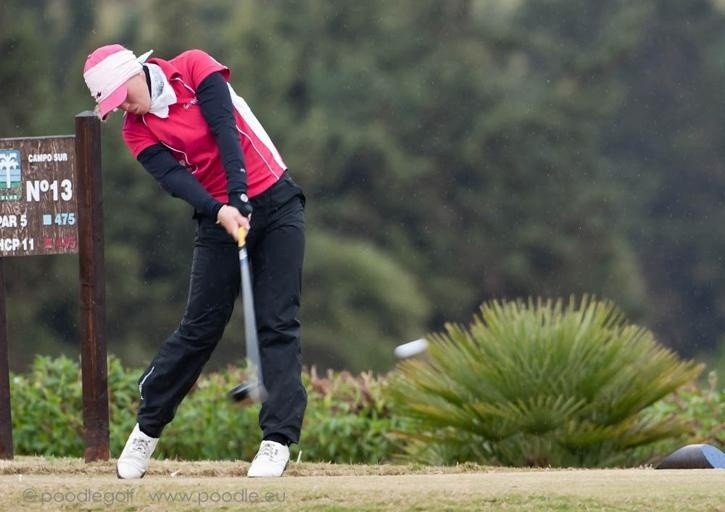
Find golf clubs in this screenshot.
[229,224,265,403]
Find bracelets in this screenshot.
[216,204,227,226]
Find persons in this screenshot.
[82,42,308,479]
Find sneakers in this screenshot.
[115,421,160,479]
[246,439,290,476]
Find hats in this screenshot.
[82,44,142,121]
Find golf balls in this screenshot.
[394,341,423,359]
